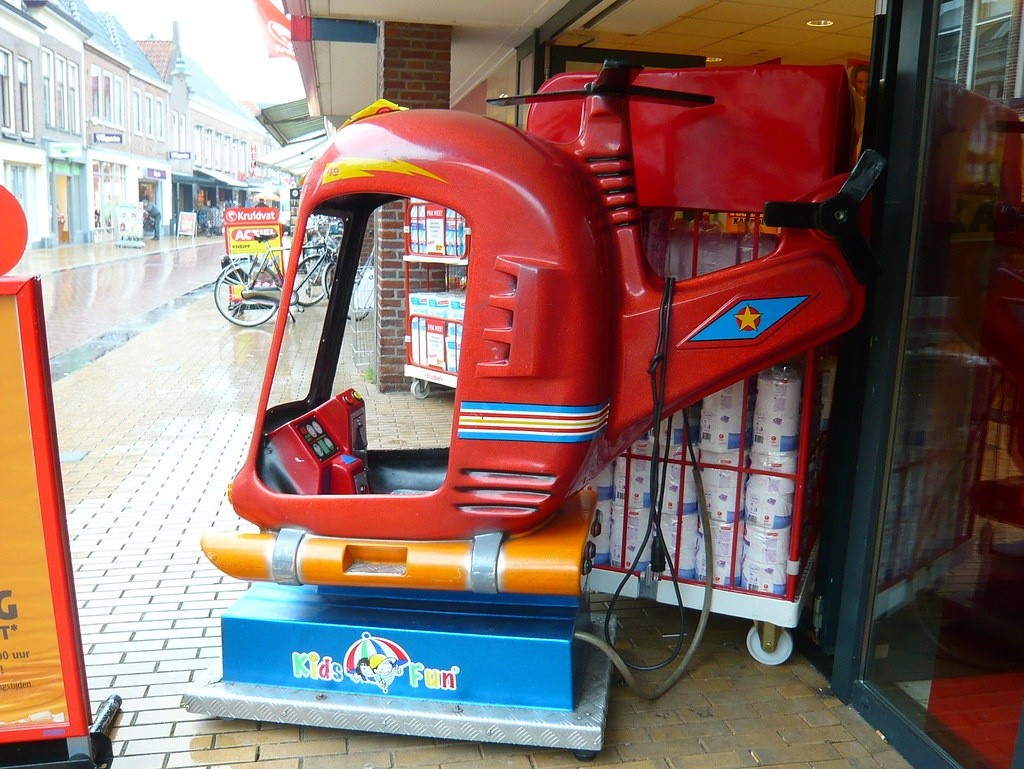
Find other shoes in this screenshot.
[152,236,159,239]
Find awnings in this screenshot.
[193,167,249,187]
[254,98,327,147]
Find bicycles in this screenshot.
[215,221,370,328]
[294,212,351,308]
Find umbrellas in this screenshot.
[255,192,280,202]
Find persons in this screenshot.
[255,199,269,207]
[142,199,161,240]
[203,200,213,236]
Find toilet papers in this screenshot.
[411,197,465,256]
[585,376,801,596]
[409,292,466,372]
